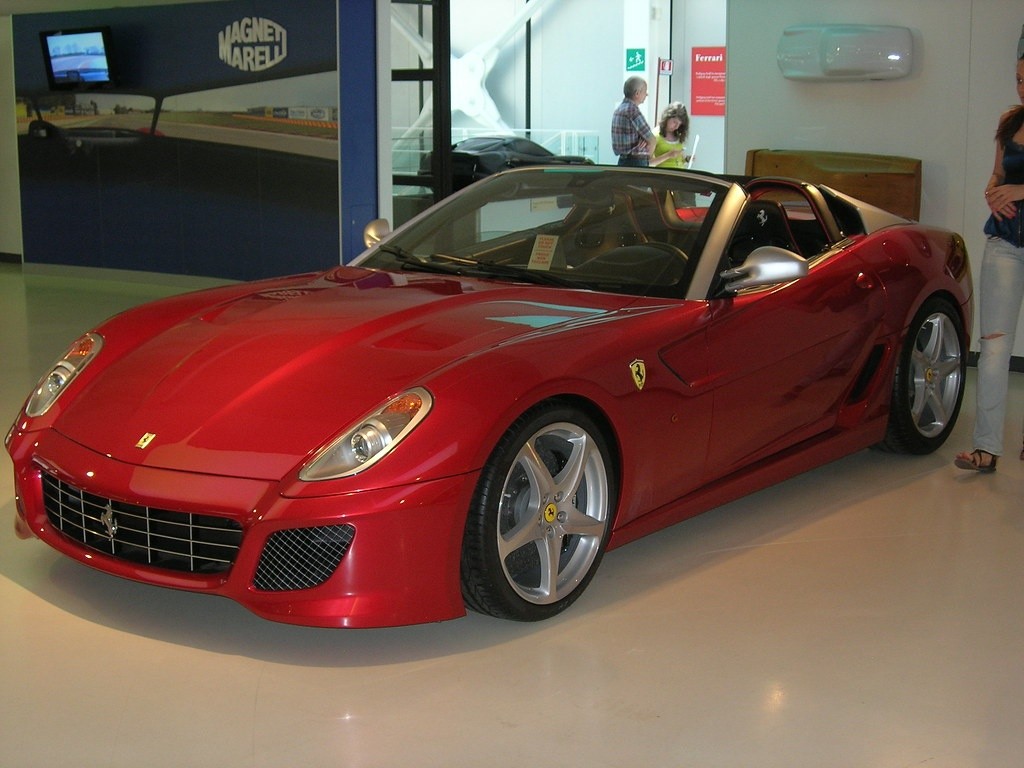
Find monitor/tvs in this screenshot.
[41,27,121,91]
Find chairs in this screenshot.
[730,199,802,261]
[548,188,648,270]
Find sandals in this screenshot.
[953,449,997,471]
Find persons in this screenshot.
[952,54,1024,472]
[611,76,656,167]
[649,102,696,168]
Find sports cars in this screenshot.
[4,164,976,630]
[417,135,596,198]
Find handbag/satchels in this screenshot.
[994,208,1022,242]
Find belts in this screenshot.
[620,155,650,160]
